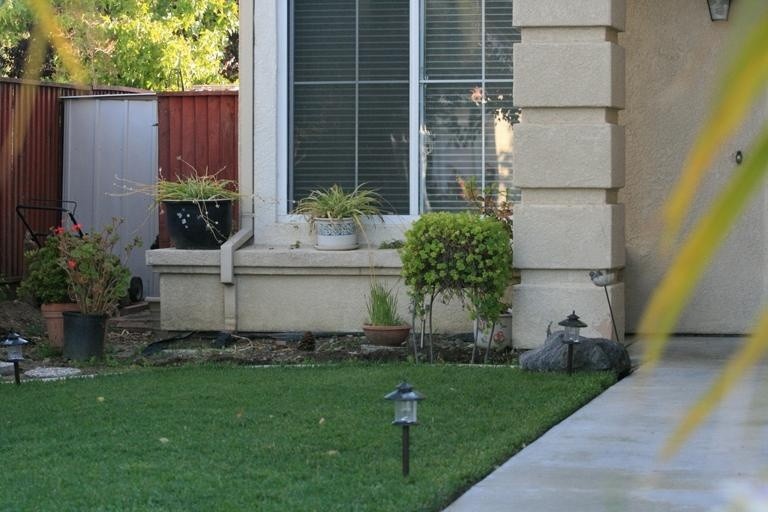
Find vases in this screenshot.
[62,310,109,364]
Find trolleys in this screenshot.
[15,197,143,303]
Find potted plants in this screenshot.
[282,181,400,250]
[14,237,83,350]
[102,155,250,249]
[362,210,521,350]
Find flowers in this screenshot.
[43,208,144,313]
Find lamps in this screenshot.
[556,309,588,375]
[707,0,732,22]
[381,377,428,480]
[3,331,30,385]
